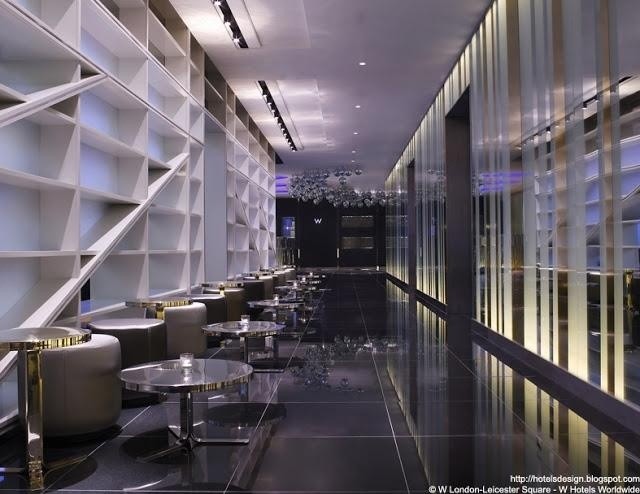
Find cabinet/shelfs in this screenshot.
[1,1,204,427]
[227,84,277,279]
[204,53,226,133]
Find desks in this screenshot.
[2,262,326,485]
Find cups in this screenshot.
[241,315,250,327]
[273,294,279,300]
[180,352,194,370]
